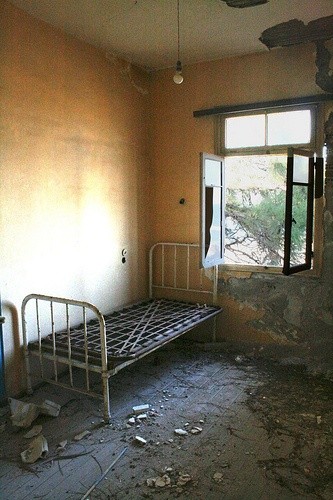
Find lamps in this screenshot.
[172,0,183,85]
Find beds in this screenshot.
[17,241,222,425]
